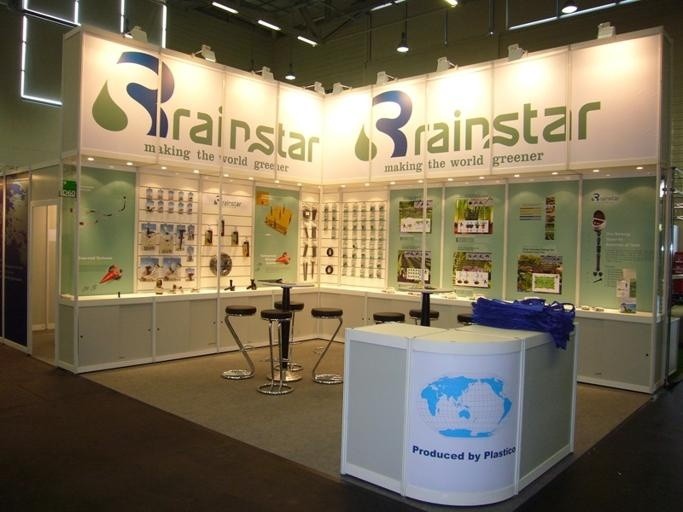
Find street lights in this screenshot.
[472,297,575,349]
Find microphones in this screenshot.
[311,308,343,385]
[275,301,304,372]
[221,305,257,380]
[410,309,439,325]
[256,309,293,395]
[458,314,474,327]
[373,312,405,325]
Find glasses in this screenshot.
[561,0,578,14]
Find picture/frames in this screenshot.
[399,286,454,327]
[261,281,314,383]
